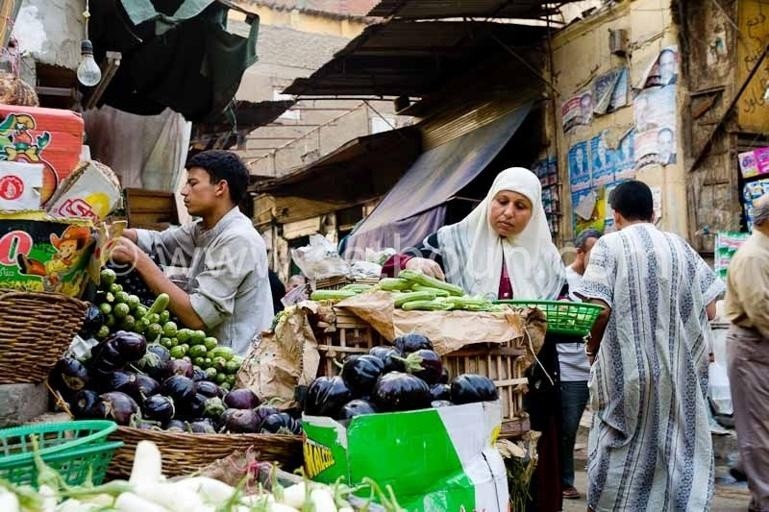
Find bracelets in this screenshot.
[584,346,598,356]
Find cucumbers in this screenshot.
[310,270,498,311]
[97,269,243,390]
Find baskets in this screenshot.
[490,300,606,337]
[0,419,124,491]
[0,288,91,384]
[306,275,380,299]
[106,425,303,482]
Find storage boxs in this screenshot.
[291,396,511,512]
[0,102,129,296]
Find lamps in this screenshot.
[74,0,104,90]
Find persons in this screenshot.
[571,180,726,511]
[579,94,591,118]
[633,95,658,135]
[556,229,602,499]
[616,134,633,162]
[287,274,306,292]
[382,166,574,511]
[571,146,588,177]
[267,267,287,316]
[656,127,676,166]
[593,139,610,169]
[724,192,769,512]
[657,49,677,86]
[112,149,271,357]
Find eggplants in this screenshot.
[49,305,303,435]
[303,332,499,426]
